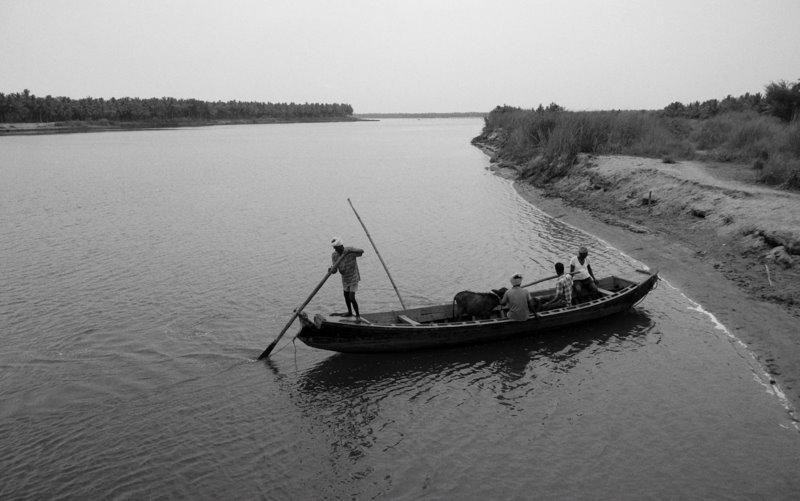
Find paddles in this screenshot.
[257,250,349,361]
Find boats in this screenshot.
[298,264,662,353]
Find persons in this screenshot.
[543,263,573,309]
[328,238,364,323]
[499,274,541,322]
[571,247,599,304]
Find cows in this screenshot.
[452,287,508,319]
